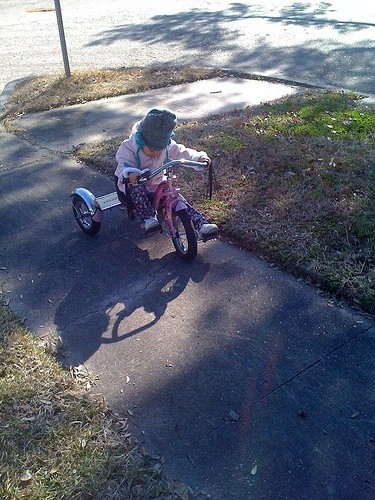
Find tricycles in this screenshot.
[67,158,198,261]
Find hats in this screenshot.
[135,108,178,152]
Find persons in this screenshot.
[113,107,219,243]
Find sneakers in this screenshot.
[199,223,219,242]
[144,218,160,232]
